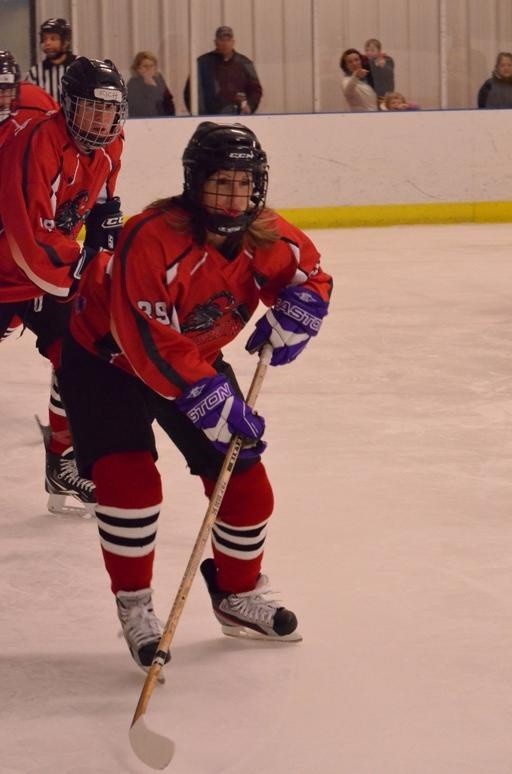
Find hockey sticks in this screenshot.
[129,344,274,770]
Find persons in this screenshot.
[475,51,511,107]
[0,55,131,503]
[362,37,394,99]
[24,15,83,104]
[338,46,379,111]
[0,48,60,145]
[57,119,336,670]
[181,23,264,115]
[380,91,421,110]
[127,49,177,118]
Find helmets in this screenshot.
[40,17,71,60]
[182,122,269,236]
[61,58,129,148]
[1,50,19,113]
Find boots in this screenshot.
[115,590,171,665]
[199,558,298,636]
[35,418,99,504]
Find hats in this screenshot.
[215,25,236,37]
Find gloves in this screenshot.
[178,373,266,459]
[73,198,123,280]
[246,288,328,367]
